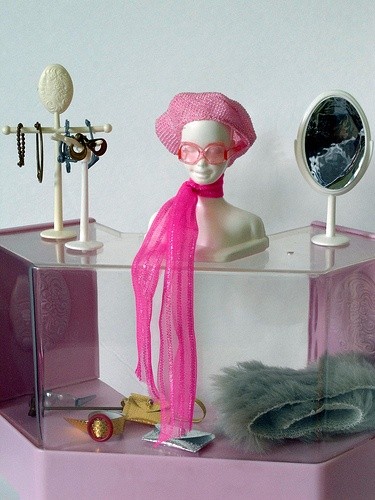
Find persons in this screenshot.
[146,92,271,265]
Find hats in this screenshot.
[151,91,257,166]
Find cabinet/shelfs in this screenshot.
[0,219,374,499]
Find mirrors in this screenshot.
[295,88,375,249]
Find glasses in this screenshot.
[175,142,246,164]
